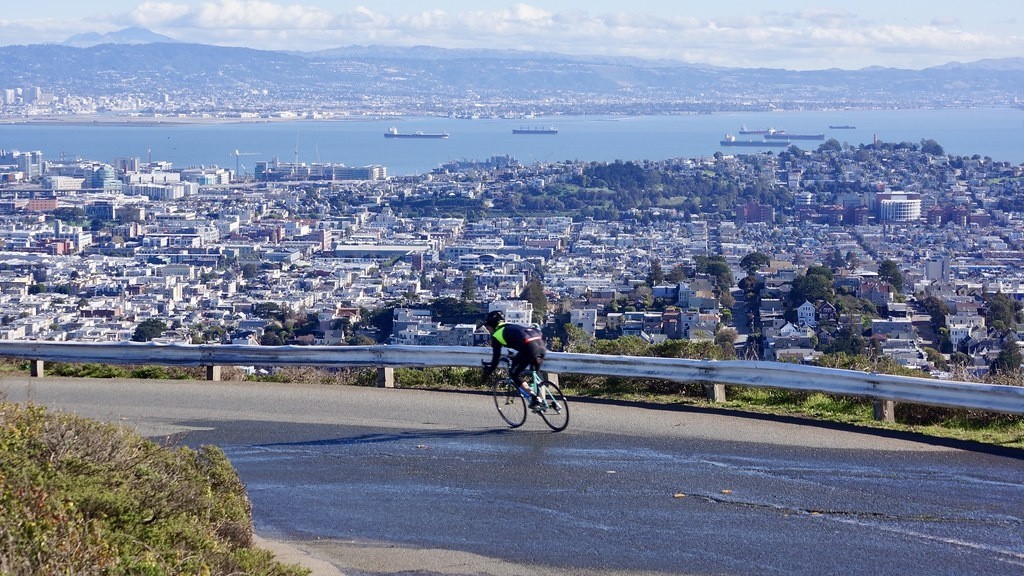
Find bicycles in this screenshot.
[481,349,570,431]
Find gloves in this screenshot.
[507,354,516,360]
[485,363,496,374]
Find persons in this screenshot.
[481,310,550,412]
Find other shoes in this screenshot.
[528,397,543,409]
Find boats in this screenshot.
[385,125,450,138]
[764,128,825,140]
[721,133,792,147]
[738,125,784,134]
[513,125,559,134]
[827,126,857,129]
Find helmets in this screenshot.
[483,310,505,329]
